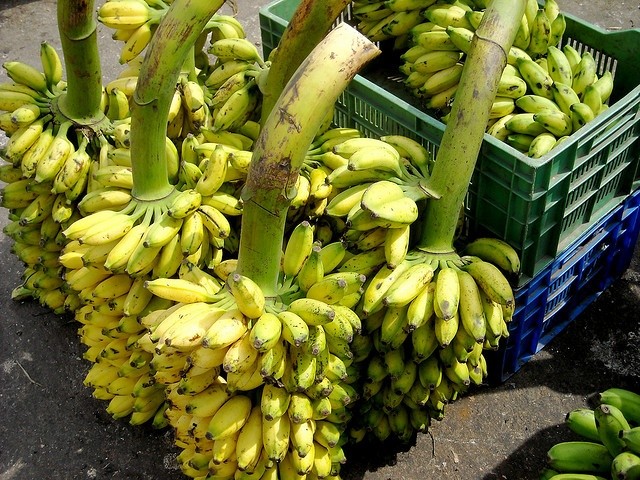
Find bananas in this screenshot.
[545,387,639,478]
[350,0,612,159]
[0,0,520,480]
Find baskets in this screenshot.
[490,189,640,383]
[259,0,640,292]
